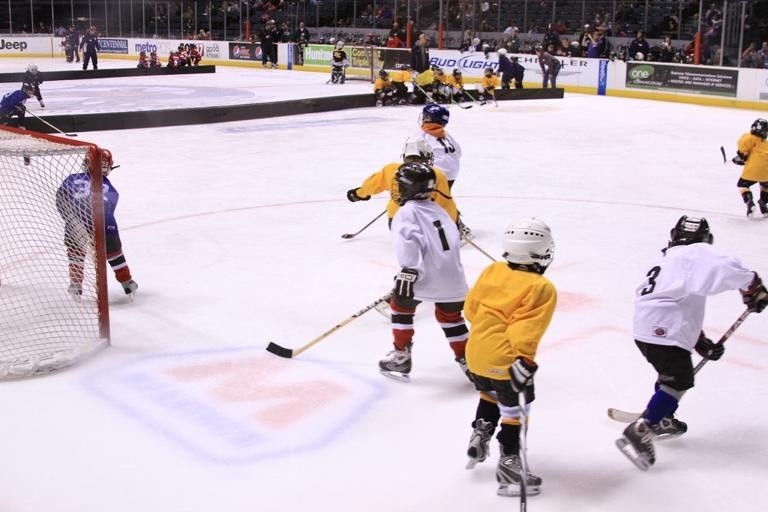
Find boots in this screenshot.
[743,199,755,215]
[479,98,486,106]
[40,100,45,107]
[378,342,414,373]
[651,414,688,435]
[457,219,471,239]
[425,96,465,104]
[496,442,542,486]
[467,417,495,462]
[116,269,138,294]
[68,283,83,295]
[624,407,656,465]
[376,96,406,107]
[453,354,480,383]
[758,199,768,213]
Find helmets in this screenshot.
[418,102,450,127]
[390,162,435,206]
[88,25,97,32]
[534,44,545,52]
[502,217,554,274]
[671,215,713,243]
[335,41,345,50]
[431,64,462,75]
[27,64,39,75]
[379,63,411,81]
[750,118,767,139]
[85,147,114,177]
[484,47,518,77]
[400,135,435,165]
[22,80,35,99]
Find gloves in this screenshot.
[508,355,537,394]
[738,154,748,165]
[733,150,744,163]
[394,268,418,297]
[347,187,371,202]
[695,330,724,360]
[739,271,767,313]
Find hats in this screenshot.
[419,34,431,41]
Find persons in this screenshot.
[137,44,201,68]
[614,211,766,473]
[149,1,298,42]
[0,82,35,131]
[56,144,140,299]
[458,215,558,502]
[343,134,458,237]
[319,1,767,109]
[262,19,309,69]
[21,21,49,34]
[22,65,45,107]
[373,158,484,388]
[416,101,472,241]
[729,113,767,215]
[62,19,102,70]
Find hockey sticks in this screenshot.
[720,146,736,164]
[25,109,79,137]
[439,89,473,109]
[341,207,390,240]
[606,310,751,425]
[463,88,487,106]
[264,289,389,359]
